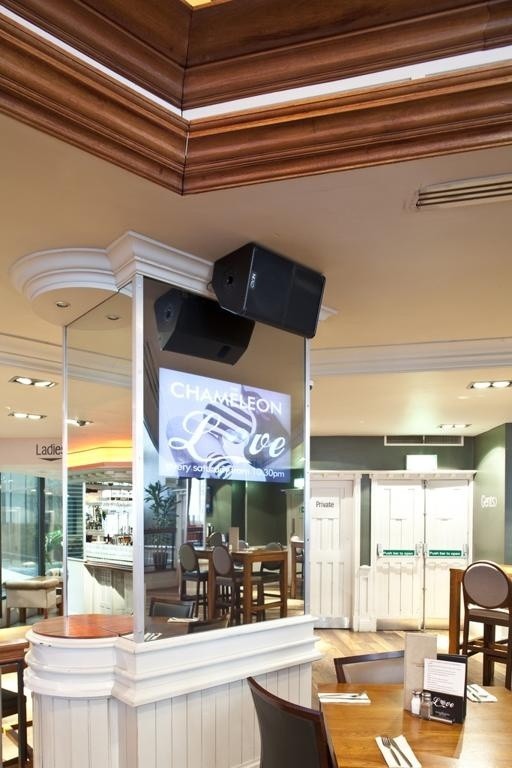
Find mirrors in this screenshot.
[66,282,134,642]
[142,276,305,641]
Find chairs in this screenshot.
[0,639,29,768]
[234,541,248,565]
[333,651,468,684]
[211,544,263,628]
[252,542,284,621]
[462,559,511,690]
[247,677,334,768]
[291,542,304,598]
[149,597,195,618]
[179,543,208,620]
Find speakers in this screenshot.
[154,286,256,366]
[212,241,326,339]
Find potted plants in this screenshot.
[144,480,184,570]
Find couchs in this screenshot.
[40,568,63,616]
[6,579,63,627]
